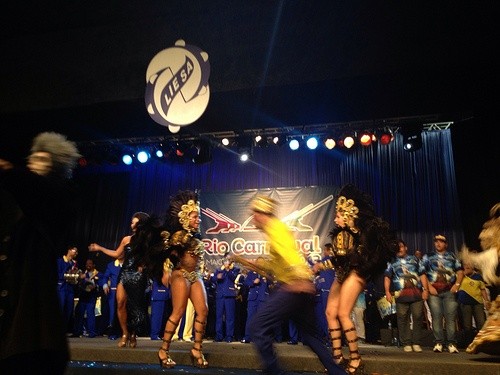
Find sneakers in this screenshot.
[433,343,443,351]
[448,345,458,353]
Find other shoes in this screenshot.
[240,339,249,343]
[413,345,422,352]
[227,340,231,343]
[110,337,116,341]
[155,337,161,340]
[404,345,412,352]
[85,334,94,338]
[287,340,293,344]
[185,338,191,342]
[171,338,174,342]
[68,333,82,338]
[213,339,219,342]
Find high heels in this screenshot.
[118,334,128,348]
[316,356,347,375]
[190,350,208,369]
[158,350,176,368]
[344,357,365,375]
[129,335,137,348]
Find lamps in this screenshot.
[323,126,393,150]
[122,138,213,166]
[216,136,238,149]
[253,132,287,145]
[401,121,425,152]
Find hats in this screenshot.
[433,234,447,242]
[249,193,280,215]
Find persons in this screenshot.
[58,243,103,337]
[312,244,335,347]
[129,189,209,369]
[461,202,500,353]
[384,240,428,352]
[418,234,464,354]
[0,131,82,375]
[151,279,170,340]
[88,212,151,348]
[102,248,128,339]
[268,257,320,345]
[228,193,349,375]
[200,252,270,342]
[414,250,422,258]
[312,185,399,375]
[456,263,490,347]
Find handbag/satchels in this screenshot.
[376,295,397,320]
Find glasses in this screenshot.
[70,249,78,254]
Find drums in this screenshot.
[376,294,398,320]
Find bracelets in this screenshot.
[423,288,428,290]
[456,283,460,286]
